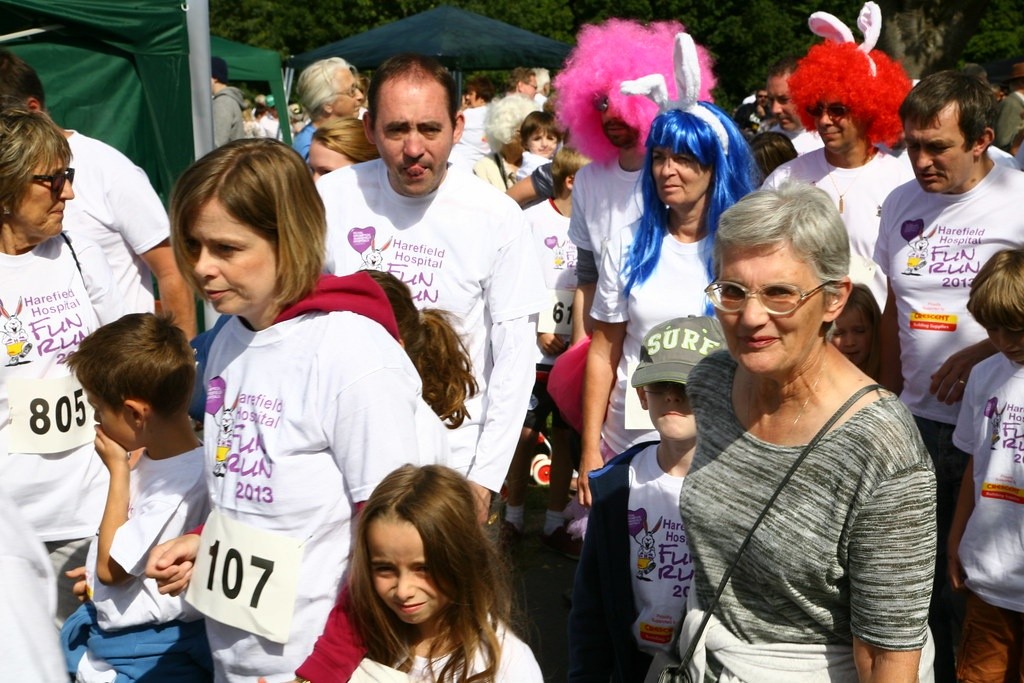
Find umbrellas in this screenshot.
[280,6,572,108]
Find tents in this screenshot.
[0,0,295,209]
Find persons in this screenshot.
[314,56,539,528]
[497,148,588,563]
[0,43,201,683]
[760,35,1024,683]
[207,54,827,211]
[553,16,937,683]
[145,139,545,683]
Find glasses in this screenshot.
[334,87,357,98]
[28,167,75,195]
[593,95,609,111]
[808,101,850,117]
[703,273,838,316]
[642,381,687,394]
[757,94,767,99]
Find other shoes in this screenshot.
[540,522,584,559]
[494,508,526,554]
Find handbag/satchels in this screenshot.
[643,648,693,683]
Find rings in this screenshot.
[958,380,967,385]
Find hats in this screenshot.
[265,95,275,108]
[211,57,228,84]
[1005,62,1024,81]
[630,314,730,389]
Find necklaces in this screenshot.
[745,353,830,444]
